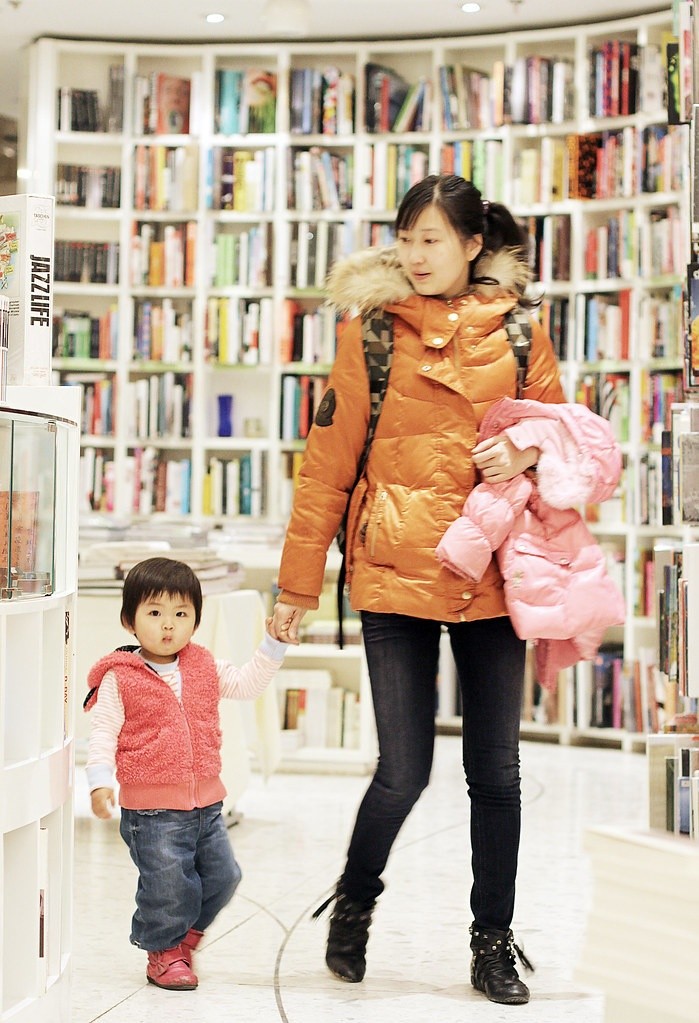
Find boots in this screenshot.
[469,921,535,1004]
[312,885,377,983]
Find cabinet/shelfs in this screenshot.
[20,0,699,855]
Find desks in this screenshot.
[74,589,266,828]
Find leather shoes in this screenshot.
[182,928,206,970]
[144,943,196,991]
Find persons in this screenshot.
[83,558,299,990]
[271,175,567,1004]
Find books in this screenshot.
[1,0,698,836]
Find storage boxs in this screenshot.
[0,193,55,385]
